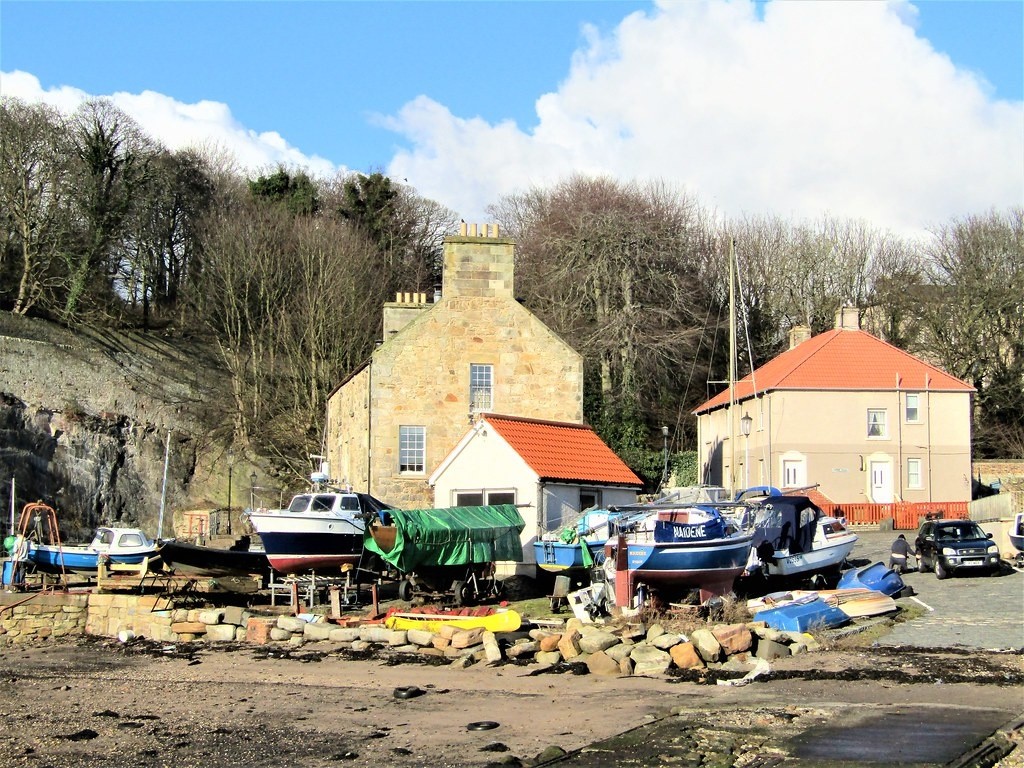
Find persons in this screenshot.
[11,530,27,591]
[889,534,922,574]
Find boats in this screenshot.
[3,426,176,569]
[152,532,287,585]
[753,599,850,633]
[384,607,522,633]
[369,511,527,569]
[1008,512,1024,552]
[601,497,753,604]
[241,424,399,577]
[526,500,640,573]
[835,561,906,600]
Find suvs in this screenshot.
[913,515,1001,580]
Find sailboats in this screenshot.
[653,237,857,583]
